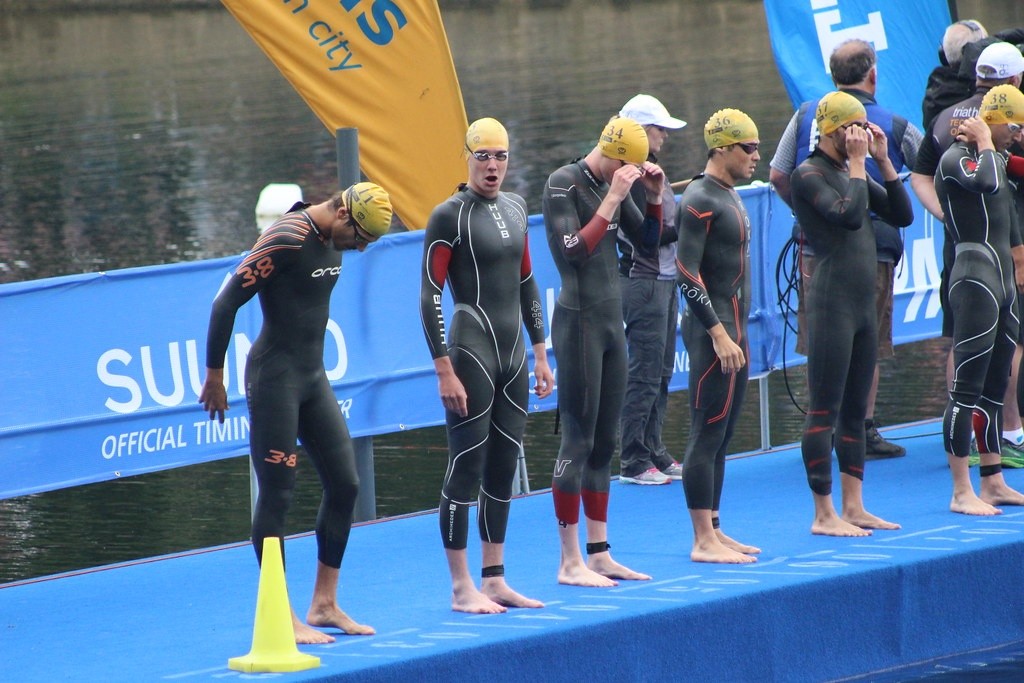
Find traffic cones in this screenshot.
[227,535,320,674]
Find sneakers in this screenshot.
[865,420,906,461]
[948,437,1024,468]
[619,460,683,484]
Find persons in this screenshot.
[420,116,556,614]
[618,94,685,485]
[768,39,924,462]
[789,90,901,538]
[673,108,762,564]
[542,117,664,589]
[199,182,393,646]
[910,20,1024,517]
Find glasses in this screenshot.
[466,144,508,161]
[738,141,759,154]
[841,121,869,131]
[1008,123,1024,136]
[350,218,370,249]
[620,160,646,180]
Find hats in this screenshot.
[704,108,759,150]
[618,94,687,130]
[598,116,648,164]
[816,91,867,137]
[975,42,1024,79]
[978,84,1024,125]
[342,182,393,237]
[463,119,510,162]
